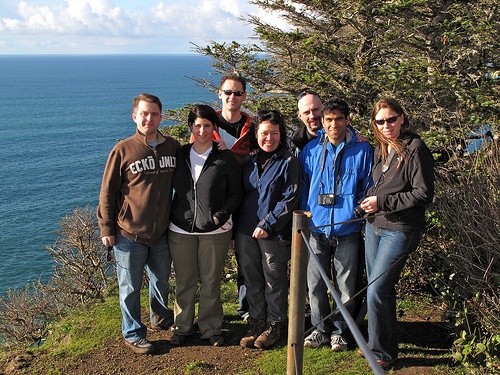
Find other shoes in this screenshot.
[169,334,188,345]
[124,337,153,354]
[209,333,225,346]
[367,352,398,368]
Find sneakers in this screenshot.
[304,329,329,348]
[254,320,282,348]
[240,327,259,347]
[330,332,348,351]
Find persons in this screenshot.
[97,93,182,355]
[290,91,367,328]
[236,109,303,348]
[169,105,244,347]
[356,97,434,370]
[298,98,374,355]
[213,75,256,323]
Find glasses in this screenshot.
[220,89,244,97]
[297,90,320,99]
[375,115,401,126]
[258,109,283,119]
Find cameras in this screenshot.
[318,193,335,205]
[354,196,368,219]
